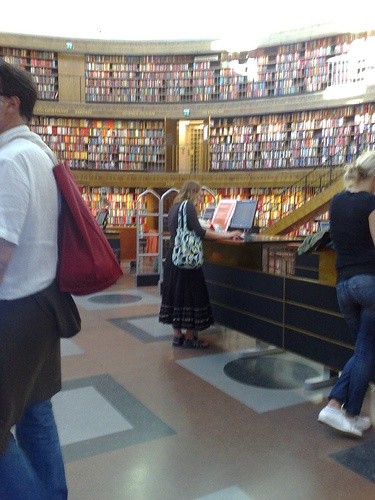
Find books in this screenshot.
[0,31,375,278]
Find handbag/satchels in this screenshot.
[173,200,204,270]
[53,159,124,294]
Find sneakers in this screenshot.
[318,407,363,439]
[340,410,371,431]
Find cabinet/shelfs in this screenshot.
[0,17,375,262]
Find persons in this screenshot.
[0,58,81,500]
[317,150,375,438]
[159,180,242,349]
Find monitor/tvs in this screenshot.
[229,200,259,234]
[96,210,109,230]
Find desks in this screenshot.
[103,231,121,266]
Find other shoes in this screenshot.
[182,336,208,347]
[173,335,184,346]
